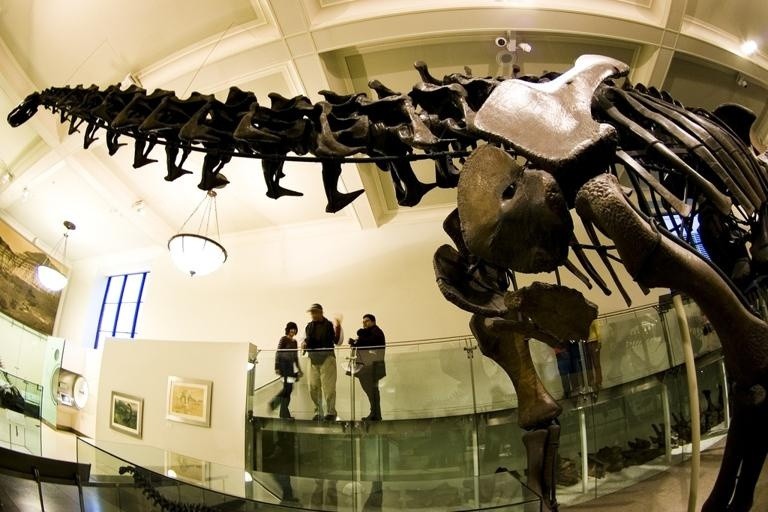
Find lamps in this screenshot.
[168,190,227,279]
[36,221,76,295]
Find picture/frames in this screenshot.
[0,216,70,339]
[164,453,211,486]
[166,377,213,429]
[109,390,144,442]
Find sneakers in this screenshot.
[313,414,338,421]
[361,415,382,421]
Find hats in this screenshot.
[307,303,323,312]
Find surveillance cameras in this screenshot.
[495,37,507,48]
[741,80,748,88]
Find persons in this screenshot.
[267,321,304,418]
[273,472,302,508]
[348,313,386,421]
[309,478,340,512]
[361,481,385,512]
[304,304,342,423]
